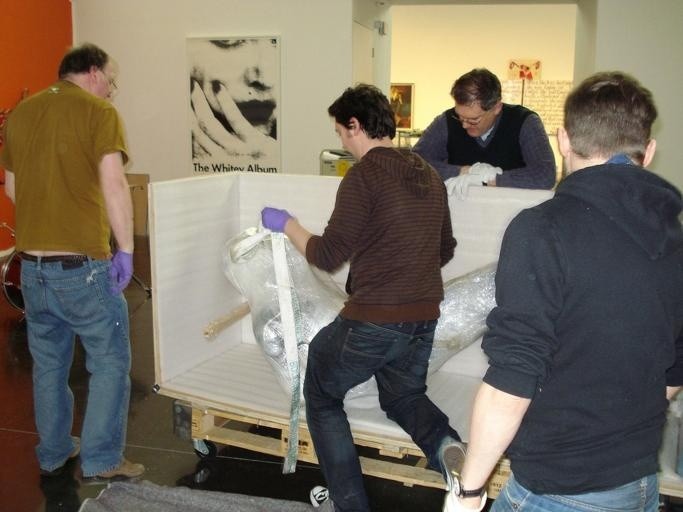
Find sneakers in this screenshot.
[310,486,335,510]
[97,459,145,479]
[439,440,487,512]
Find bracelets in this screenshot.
[482,180,489,187]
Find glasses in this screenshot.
[101,67,119,96]
[452,112,486,126]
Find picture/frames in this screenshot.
[182,30,285,176]
[389,82,416,130]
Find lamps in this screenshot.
[505,58,542,107]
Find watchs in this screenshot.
[447,473,488,500]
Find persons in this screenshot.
[187,36,278,162]
[261,84,466,509]
[410,66,558,200]
[37,470,133,512]
[0,41,147,480]
[432,69,683,510]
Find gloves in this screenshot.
[108,250,133,296]
[444,162,503,200]
[261,207,290,232]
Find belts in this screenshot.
[19,251,94,264]
